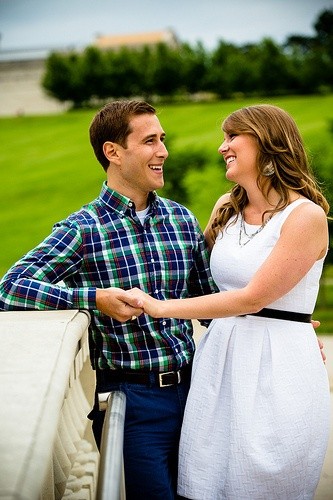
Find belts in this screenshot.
[97,365,192,388]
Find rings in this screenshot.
[131,315,137,320]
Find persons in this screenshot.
[126,105,330,500]
[0,100,327,500]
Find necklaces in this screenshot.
[239,194,283,248]
